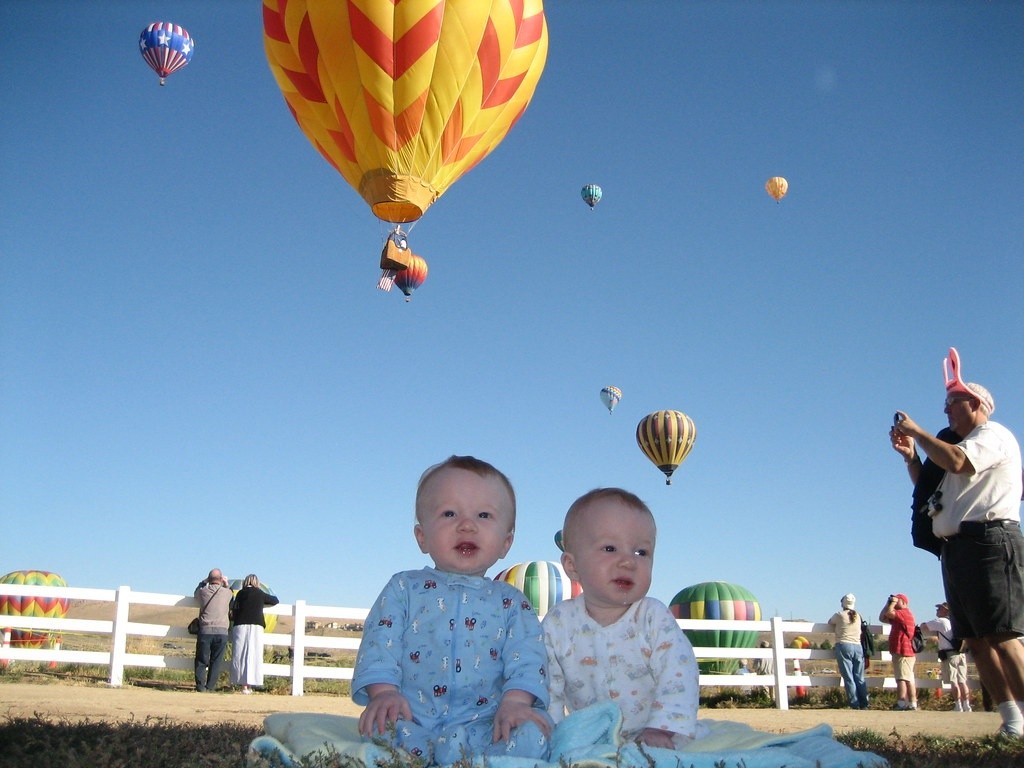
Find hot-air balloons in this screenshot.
[261,0,549,270]
[226,579,279,643]
[766,175,788,207]
[666,581,762,676]
[388,255,428,303]
[789,635,811,651]
[0,570,71,651]
[139,21,195,85]
[634,410,698,485]
[582,183,604,215]
[491,530,582,622]
[598,385,622,415]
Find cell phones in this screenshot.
[894,413,900,427]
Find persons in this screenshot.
[920,602,972,712]
[231,574,279,695]
[888,382,1024,739]
[733,659,752,695]
[351,456,555,768]
[194,568,234,692]
[542,487,699,750]
[400,237,406,249]
[828,594,869,710]
[879,594,918,710]
[754,641,776,700]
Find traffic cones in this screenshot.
[934,673,943,696]
[795,664,805,696]
[47,637,60,671]
[1,628,12,670]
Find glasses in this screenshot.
[945,395,977,408]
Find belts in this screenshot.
[940,520,1021,542]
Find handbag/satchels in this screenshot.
[187,618,199,634]
[860,615,875,657]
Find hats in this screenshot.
[890,594,908,604]
[935,602,949,610]
[841,593,856,610]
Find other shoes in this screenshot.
[889,702,908,710]
[962,705,972,713]
[907,706,918,710]
[243,689,252,693]
[952,706,964,712]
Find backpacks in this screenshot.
[939,616,969,653]
[899,620,926,653]
